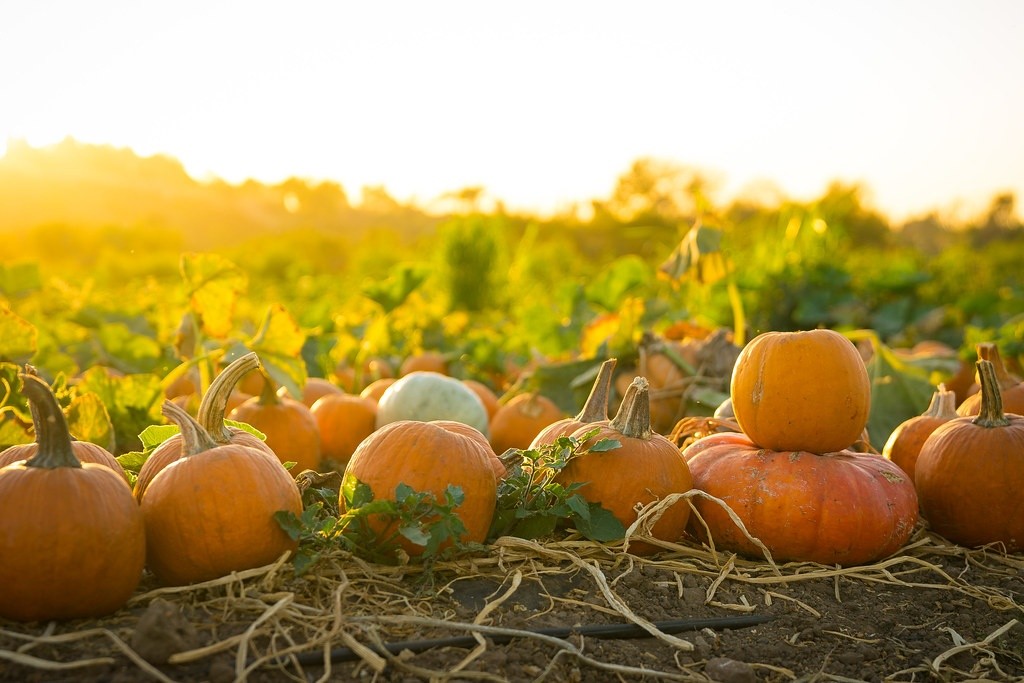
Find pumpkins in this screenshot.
[134,326,1023,584]
[1,363,147,623]
